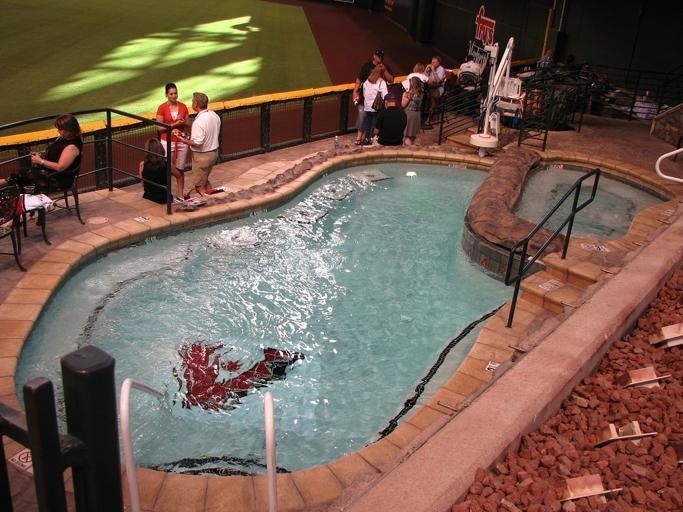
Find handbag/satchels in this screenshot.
[372,92,385,111]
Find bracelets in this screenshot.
[38,159,44,167]
[182,139,187,143]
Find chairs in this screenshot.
[0,152,86,273]
[431,68,608,134]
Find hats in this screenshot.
[384,94,396,99]
[375,49,384,56]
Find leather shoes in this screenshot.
[174,196,185,204]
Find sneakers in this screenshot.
[362,138,372,145]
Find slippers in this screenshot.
[355,139,361,145]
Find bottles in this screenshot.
[333,135,341,151]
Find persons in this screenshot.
[534,50,658,123]
[169,92,221,196]
[350,49,485,149]
[138,137,181,207]
[0,113,84,197]
[155,81,190,200]
